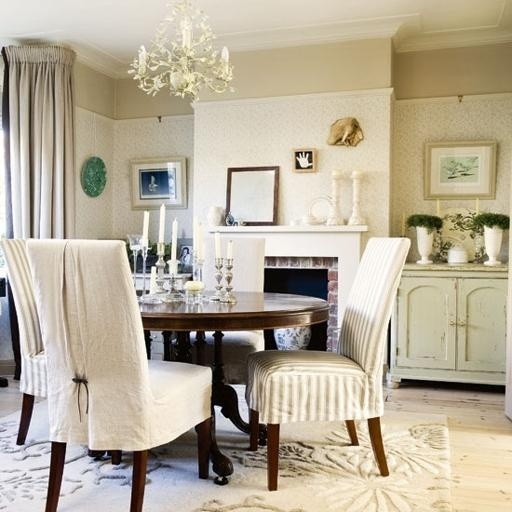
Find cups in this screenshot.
[185,290,202,305]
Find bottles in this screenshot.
[225,212,234,225]
[473,233,483,264]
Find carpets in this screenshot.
[0,384,451,512]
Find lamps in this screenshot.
[126,0,234,99]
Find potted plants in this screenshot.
[475,213,510,265]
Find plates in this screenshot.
[309,197,333,223]
[80,157,107,198]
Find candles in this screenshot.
[150,265,157,292]
[476,197,479,214]
[402,212,406,236]
[407,214,442,264]
[142,203,233,258]
[437,198,439,216]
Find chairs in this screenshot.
[25,239,212,512]
[0,239,47,445]
[171,235,266,423]
[245,237,411,490]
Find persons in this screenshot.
[179,247,190,266]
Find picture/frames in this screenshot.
[176,238,193,273]
[424,140,497,200]
[225,166,279,226]
[291,148,315,173]
[129,156,187,211]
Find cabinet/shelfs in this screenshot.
[386,263,509,388]
